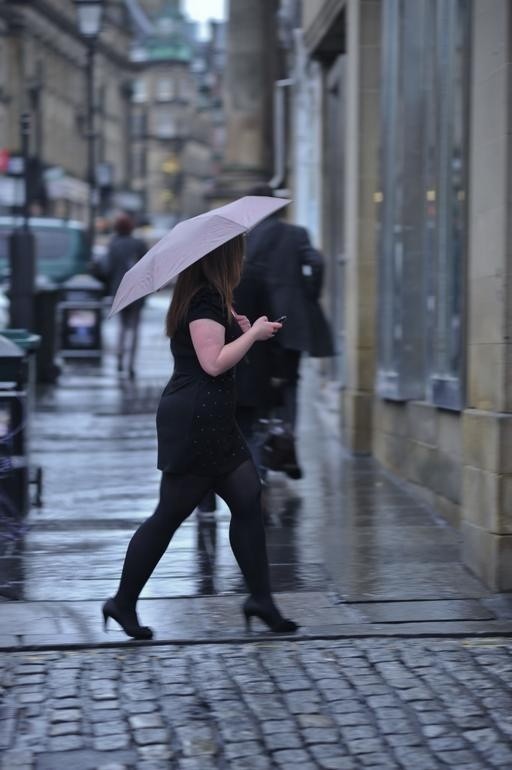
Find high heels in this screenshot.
[101,594,157,641]
[242,596,301,635]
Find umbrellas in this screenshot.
[108,195,293,319]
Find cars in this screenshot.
[0,215,95,291]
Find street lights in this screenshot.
[72,0,106,262]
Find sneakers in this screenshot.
[195,505,219,521]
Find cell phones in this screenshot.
[272,315,287,324]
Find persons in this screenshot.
[101,232,299,639]
[232,182,337,479]
[108,212,149,376]
